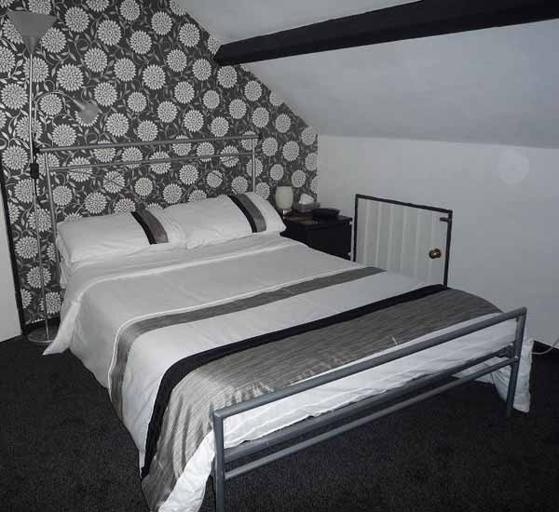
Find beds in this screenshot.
[38,130,527,511]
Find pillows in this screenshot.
[163,190,287,249]
[53,204,185,266]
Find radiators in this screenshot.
[353,194,453,286]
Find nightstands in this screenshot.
[279,210,353,261]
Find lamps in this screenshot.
[8,10,64,344]
